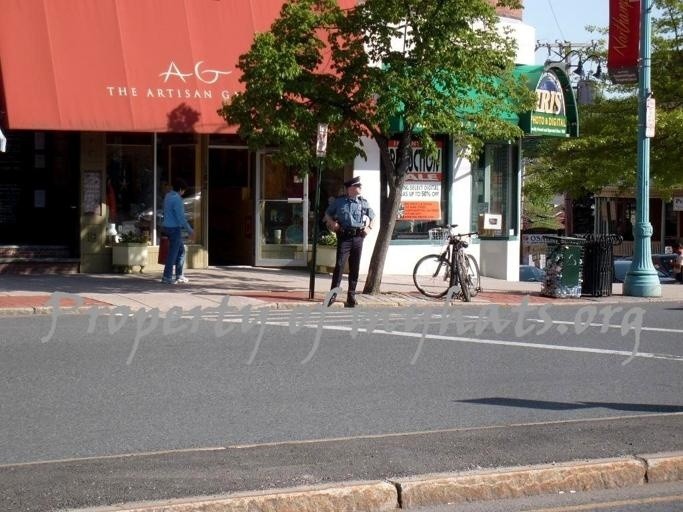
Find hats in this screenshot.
[344,175,362,187]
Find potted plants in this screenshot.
[315,233,338,274]
[112,230,151,274]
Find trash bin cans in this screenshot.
[573,232,623,297]
[541,235,586,298]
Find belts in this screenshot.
[339,225,365,237]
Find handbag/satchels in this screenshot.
[157,235,172,265]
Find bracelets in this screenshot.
[367,226,372,230]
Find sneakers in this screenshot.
[346,291,359,307]
[177,276,189,283]
[162,278,179,285]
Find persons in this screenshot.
[160,179,193,284]
[324,176,376,308]
[668,239,683,285]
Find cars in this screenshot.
[674,247,683,282]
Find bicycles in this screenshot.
[413,224,481,302]
